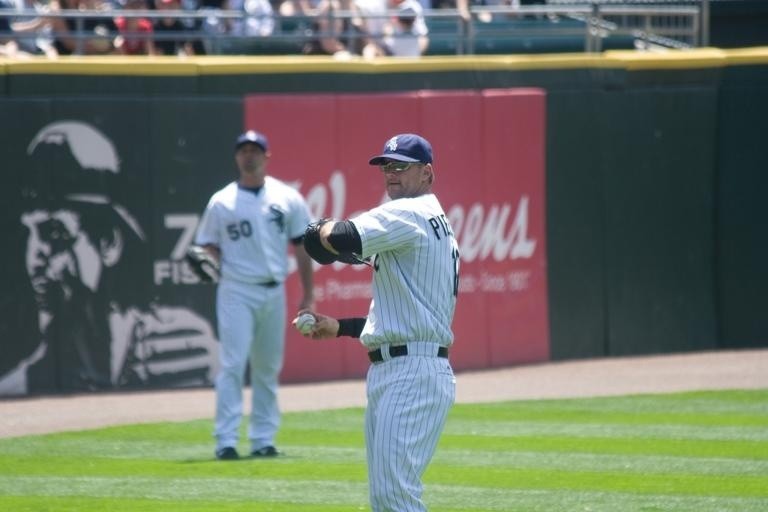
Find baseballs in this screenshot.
[297,313,317,336]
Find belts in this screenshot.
[365,344,451,366]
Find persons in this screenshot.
[1,116,219,399]
[1,0,433,61]
[185,126,320,459]
[289,130,463,512]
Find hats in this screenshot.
[366,132,436,165]
[231,129,270,154]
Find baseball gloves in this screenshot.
[187,246,221,283]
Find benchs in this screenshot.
[424,15,635,54]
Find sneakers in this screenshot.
[250,445,281,458]
[214,446,241,460]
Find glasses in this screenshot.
[378,160,427,172]
